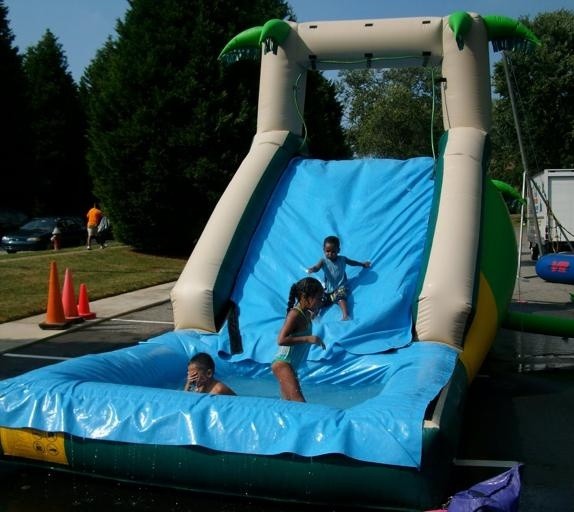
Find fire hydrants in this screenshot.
[50,227,62,251]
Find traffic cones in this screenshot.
[38,261,96,329]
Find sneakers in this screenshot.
[86,244,108,250]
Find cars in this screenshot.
[1,214,88,254]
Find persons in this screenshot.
[305,236,372,321]
[85,202,110,249]
[185,353,236,395]
[270,276,327,401]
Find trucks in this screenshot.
[526,168,574,260]
